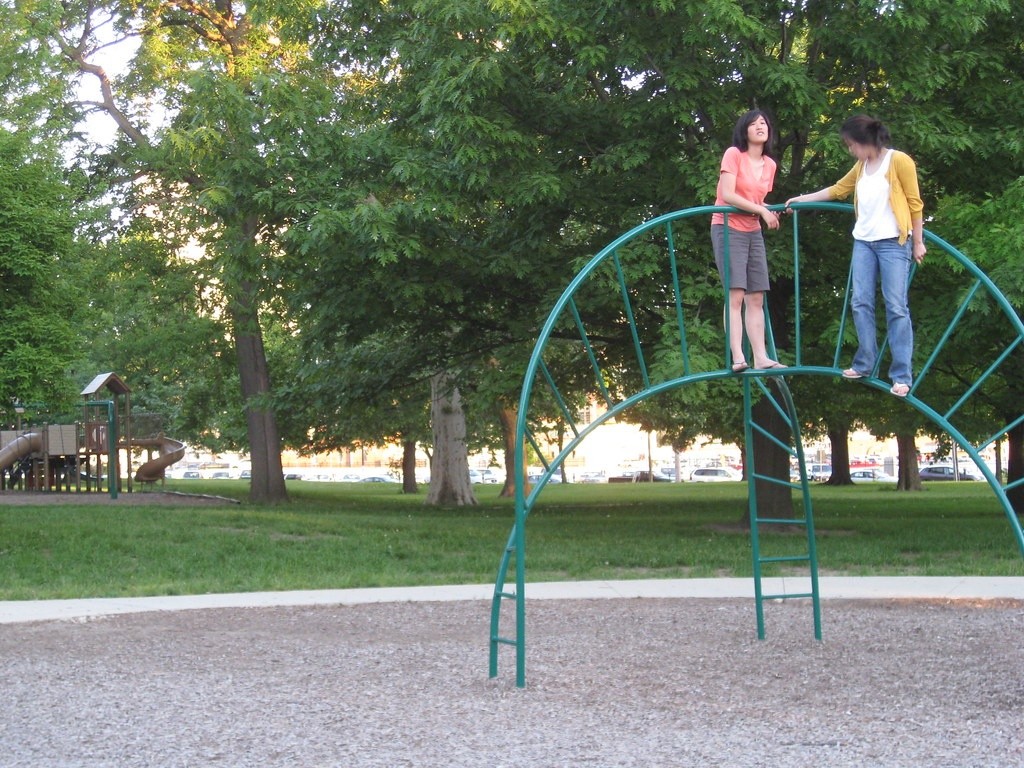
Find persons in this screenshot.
[711,110,781,373]
[779,114,927,397]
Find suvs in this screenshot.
[424,468,483,484]
[690,467,742,482]
[478,469,499,484]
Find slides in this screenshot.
[0,432,42,470]
[71,470,104,482]
[121,436,185,481]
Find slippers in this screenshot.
[889,382,910,397]
[765,361,788,369]
[841,368,869,380]
[731,361,751,374]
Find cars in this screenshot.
[849,470,893,482]
[285,473,399,483]
[529,467,604,485]
[919,466,980,482]
[790,464,832,482]
[182,462,252,481]
